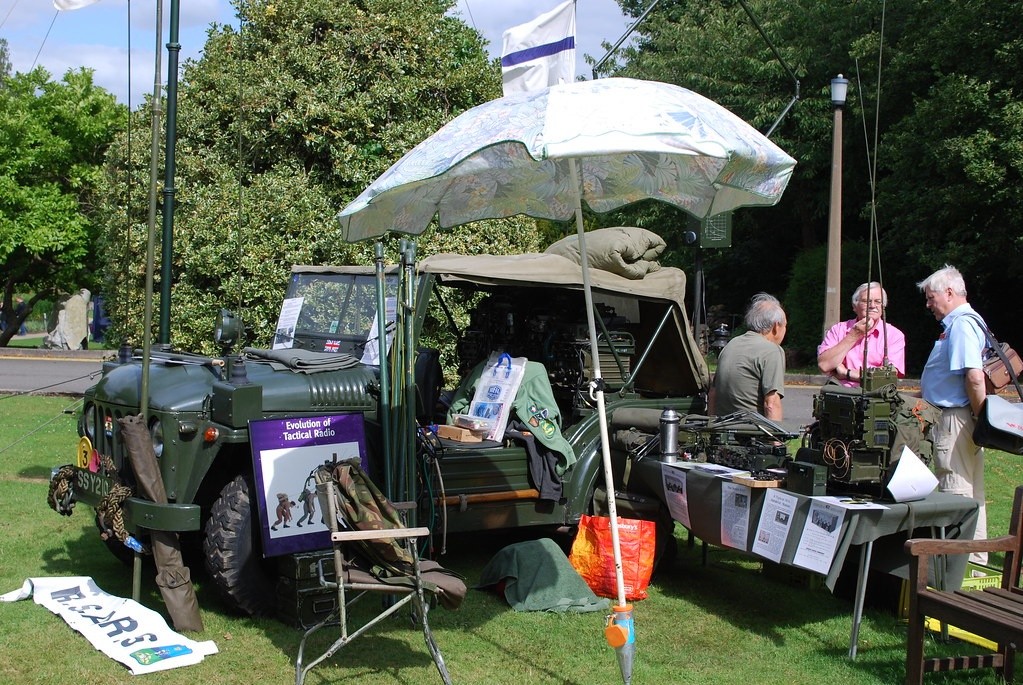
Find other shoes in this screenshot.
[271,526,278,531]
[283,525,290,528]
[308,521,314,524]
[297,522,302,527]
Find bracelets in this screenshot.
[847,369,851,380]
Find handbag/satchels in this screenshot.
[973,395,1023,456]
[593,485,675,578]
[982,341,1023,395]
[468,353,525,443]
[568,514,658,602]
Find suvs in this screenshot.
[52,257,712,627]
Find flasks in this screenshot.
[658,405,680,463]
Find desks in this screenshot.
[626,451,977,664]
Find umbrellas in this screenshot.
[336,78,798,608]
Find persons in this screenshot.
[14,296,26,336]
[708,293,787,447]
[818,281,905,388]
[916,268,991,565]
[0,301,7,331]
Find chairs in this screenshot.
[414,347,445,425]
[295,466,467,685]
[903,484,1023,685]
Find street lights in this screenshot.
[822,74,850,345]
